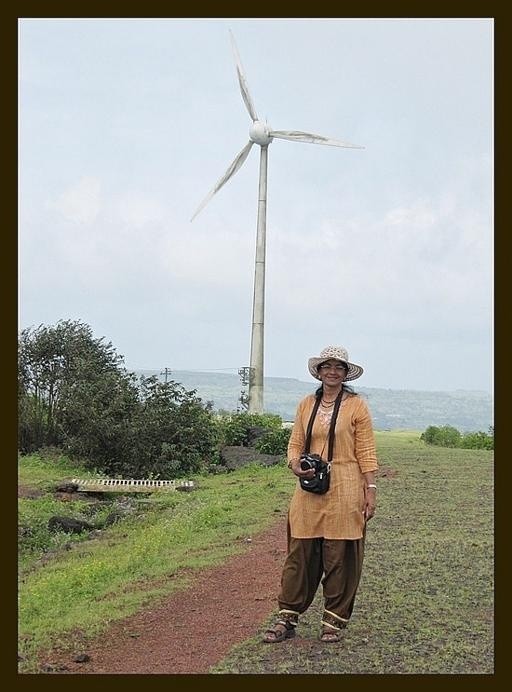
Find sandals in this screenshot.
[260,619,296,642]
[317,622,342,643]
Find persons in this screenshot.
[260,344,382,646]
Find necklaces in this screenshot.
[320,395,337,404]
[320,402,334,408]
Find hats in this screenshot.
[307,344,364,382]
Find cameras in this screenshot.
[299,454,332,494]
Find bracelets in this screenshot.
[365,481,379,490]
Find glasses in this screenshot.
[318,365,348,371]
